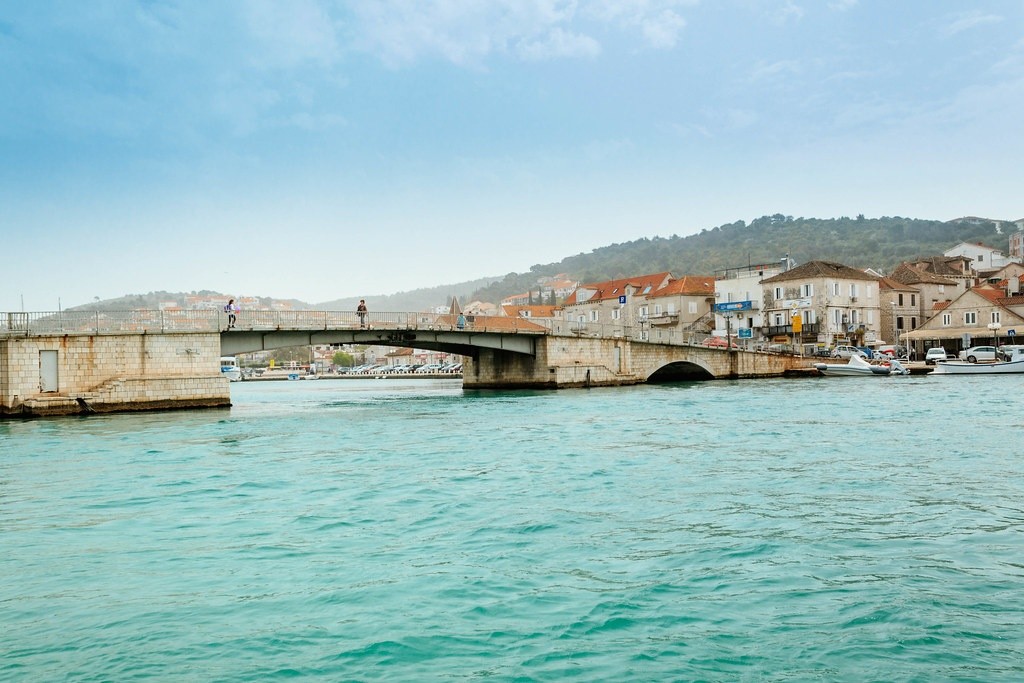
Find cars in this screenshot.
[997,344,1016,362]
[702,335,738,349]
[768,342,801,356]
[926,347,947,364]
[830,344,867,359]
[336,362,461,374]
[959,344,999,365]
[878,344,906,357]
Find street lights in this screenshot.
[704,282,720,336]
[889,301,899,361]
[289,350,293,375]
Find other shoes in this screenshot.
[232,325,236,328]
[228,325,231,328]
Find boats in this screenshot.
[926,361,1024,374]
[261,365,307,380]
[815,354,890,377]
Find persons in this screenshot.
[456,309,476,331]
[355,300,368,328]
[224,299,237,328]
[909,345,917,362]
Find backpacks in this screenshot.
[224,304,229,313]
